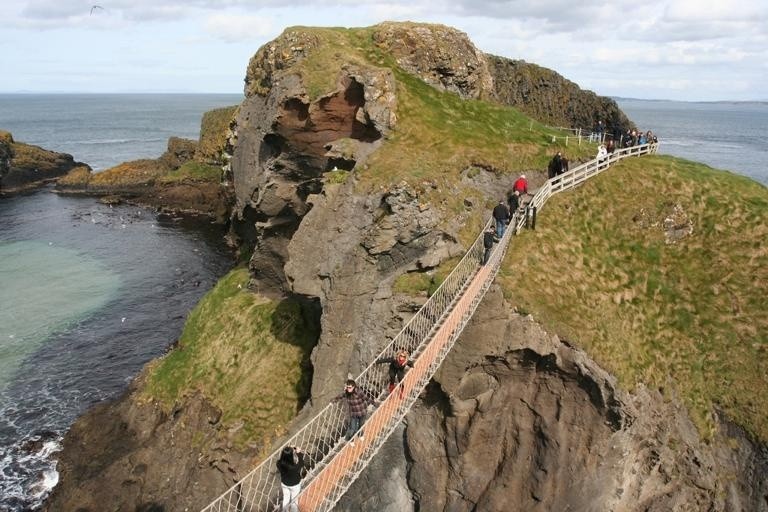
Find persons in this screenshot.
[512,174,528,196]
[595,127,657,170]
[507,191,520,225]
[492,200,508,239]
[483,225,500,265]
[375,352,414,399]
[329,379,379,446]
[550,151,569,186]
[275,445,306,512]
